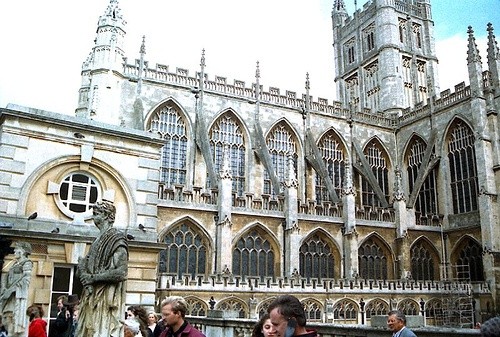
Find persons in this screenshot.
[0,239,35,336]
[123,295,207,337]
[251,293,319,336]
[387,310,417,337]
[72,197,130,337]
[26,294,80,337]
[0,315,9,337]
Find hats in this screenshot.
[63,294,80,305]
